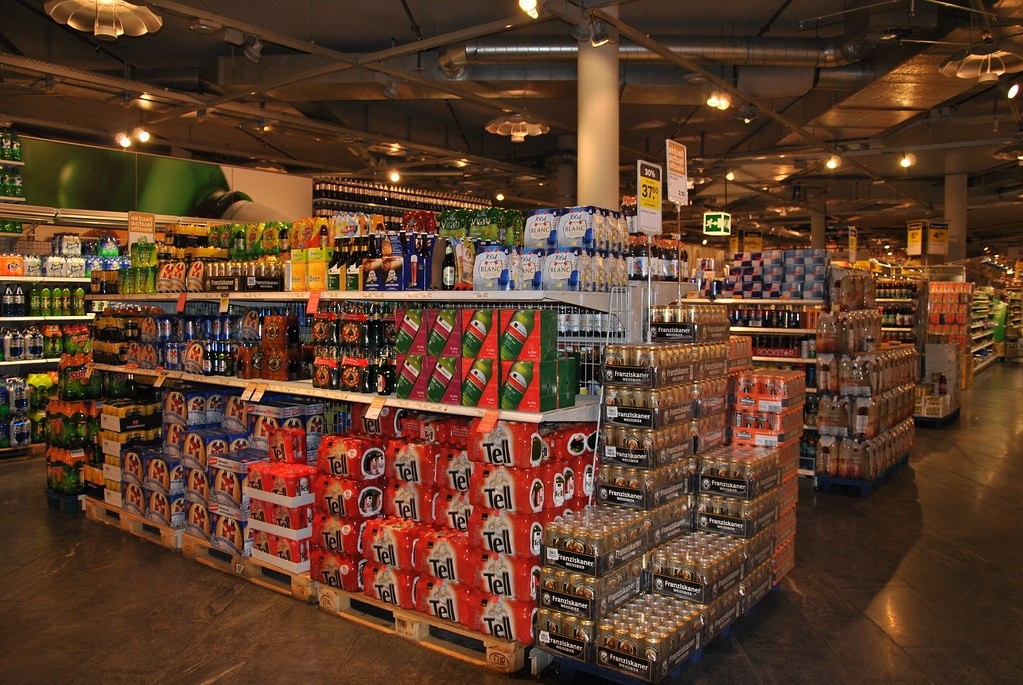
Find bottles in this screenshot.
[725,277,918,481]
[0,129,455,500]
[426,358,456,403]
[454,207,688,399]
[501,361,534,410]
[500,310,536,360]
[462,309,494,358]
[462,358,493,407]
[427,309,457,356]
[394,309,423,354]
[939,373,947,395]
[396,356,423,399]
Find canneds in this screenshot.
[204,260,284,278]
[689,257,728,296]
[129,301,312,381]
[89,242,158,295]
[535,305,806,664]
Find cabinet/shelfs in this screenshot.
[673,299,824,493]
[970,287,1023,378]
[874,298,918,331]
[84,280,641,435]
[0,275,97,464]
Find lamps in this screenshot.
[484,113,551,143]
[939,42,1023,99]
[706,88,732,110]
[993,144,1023,166]
[589,19,610,48]
[115,126,152,147]
[43,0,164,43]
[734,104,760,125]
[244,32,264,63]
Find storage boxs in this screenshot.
[202,255,405,292]
[533,319,804,685]
[686,249,832,300]
[15,241,53,258]
[173,234,209,249]
[99,410,162,509]
